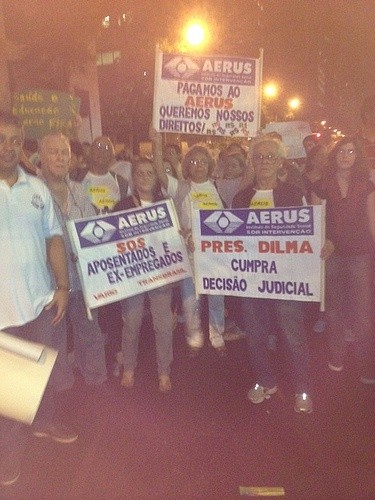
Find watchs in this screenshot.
[56,286,72,293]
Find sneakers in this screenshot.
[292,393,313,415]
[247,383,279,404]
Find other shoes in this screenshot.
[359,368,375,384]
[313,319,325,332]
[217,345,230,358]
[121,369,135,385]
[224,326,246,341]
[114,352,125,367]
[186,346,202,359]
[328,356,343,371]
[0,449,21,486]
[344,329,355,342]
[159,376,172,392]
[32,424,78,444]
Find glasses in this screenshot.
[189,159,208,165]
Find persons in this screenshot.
[21,131,374,386]
[0,113,79,486]
[188,138,339,414]
